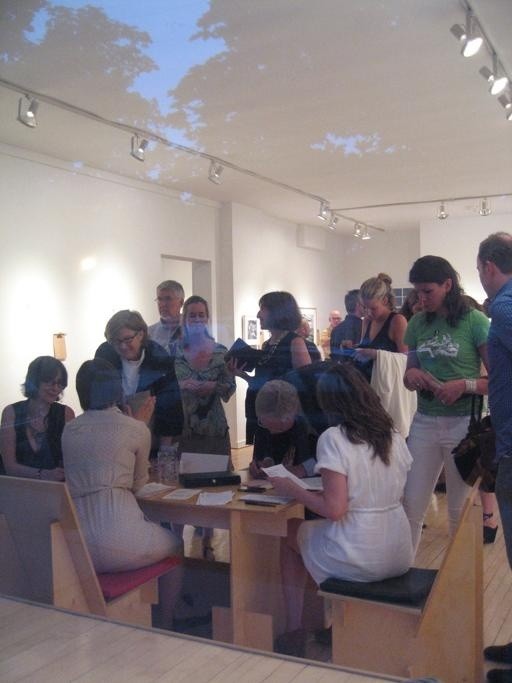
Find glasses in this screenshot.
[331,316,342,321]
[109,329,141,348]
[154,295,180,305]
[257,415,289,432]
[37,379,66,390]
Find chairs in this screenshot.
[314,472,489,682]
[1,471,189,633]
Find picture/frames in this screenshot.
[242,312,262,346]
[297,305,319,347]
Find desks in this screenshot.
[1,592,433,682]
[134,467,325,654]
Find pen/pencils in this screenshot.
[244,500,275,507]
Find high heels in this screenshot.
[274,627,307,660]
[314,624,333,646]
[483,512,499,543]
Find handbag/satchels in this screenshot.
[223,337,266,373]
[450,375,499,495]
[342,345,376,367]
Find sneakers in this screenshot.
[483,641,511,682]
[172,610,209,632]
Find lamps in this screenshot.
[322,202,374,241]
[446,6,511,124]
[432,191,491,221]
[14,87,330,220]
